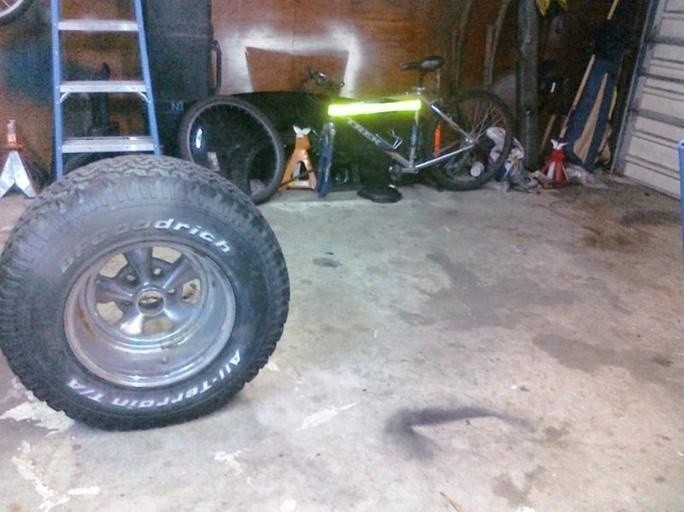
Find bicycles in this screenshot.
[300,57,513,200]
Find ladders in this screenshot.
[51,0,161,180]
